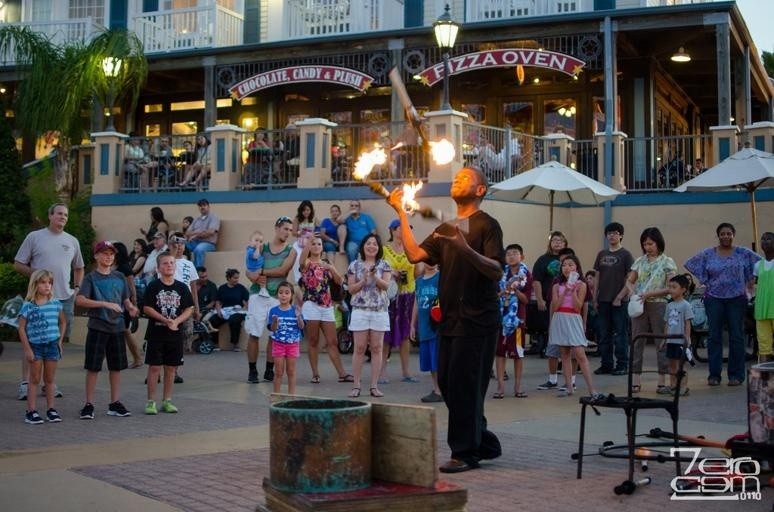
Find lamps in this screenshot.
[557,106,577,118]
[669,43,692,63]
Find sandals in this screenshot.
[728,376,742,386]
[242,369,441,403]
[493,365,716,400]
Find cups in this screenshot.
[566,271,581,289]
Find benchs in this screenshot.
[68,217,351,358]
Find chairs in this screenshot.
[575,333,688,486]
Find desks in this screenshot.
[145,155,184,192]
[247,146,292,187]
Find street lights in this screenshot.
[97,46,125,134]
[426,3,465,112]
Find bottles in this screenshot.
[506,276,524,291]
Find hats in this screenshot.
[388,218,413,231]
[94,240,118,254]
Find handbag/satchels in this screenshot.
[330,276,346,302]
[627,295,645,318]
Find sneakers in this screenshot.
[159,399,178,413]
[18,379,29,400]
[45,408,62,422]
[80,402,95,419]
[24,409,45,424]
[144,398,158,415]
[41,384,63,398]
[107,400,131,417]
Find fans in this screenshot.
[550,98,577,111]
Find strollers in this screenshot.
[336,281,393,360]
[142,309,223,357]
